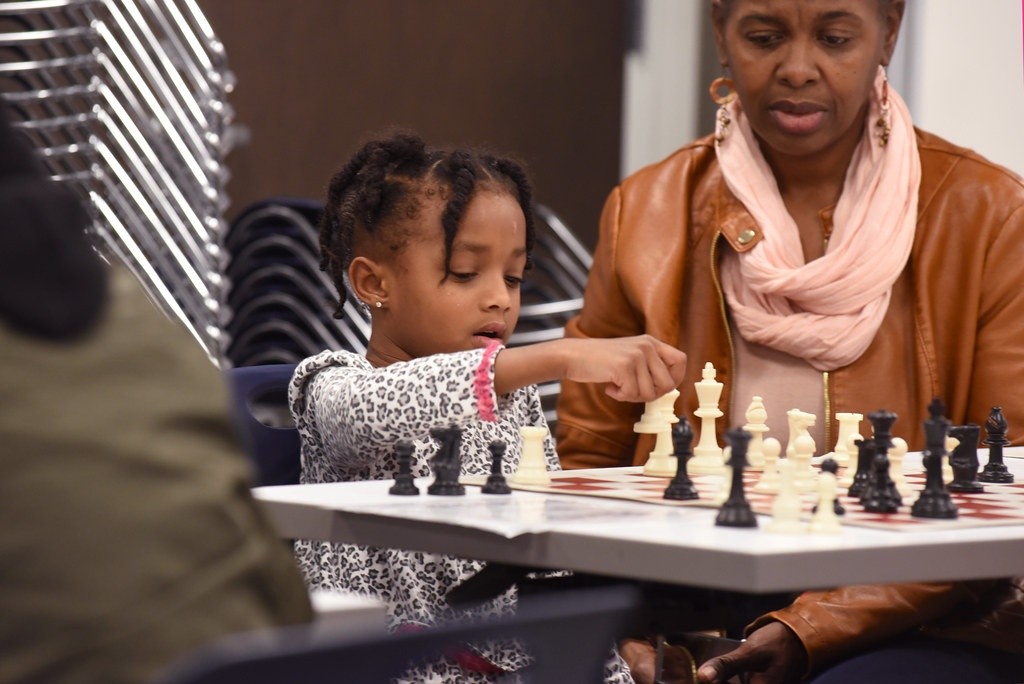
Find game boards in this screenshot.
[457,466,1024,532]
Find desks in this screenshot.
[246,444,1024,598]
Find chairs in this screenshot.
[225,362,308,489]
[157,586,637,683]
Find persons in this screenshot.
[0,93,311,684]
[289,128,688,684]
[552,0,1024,684]
